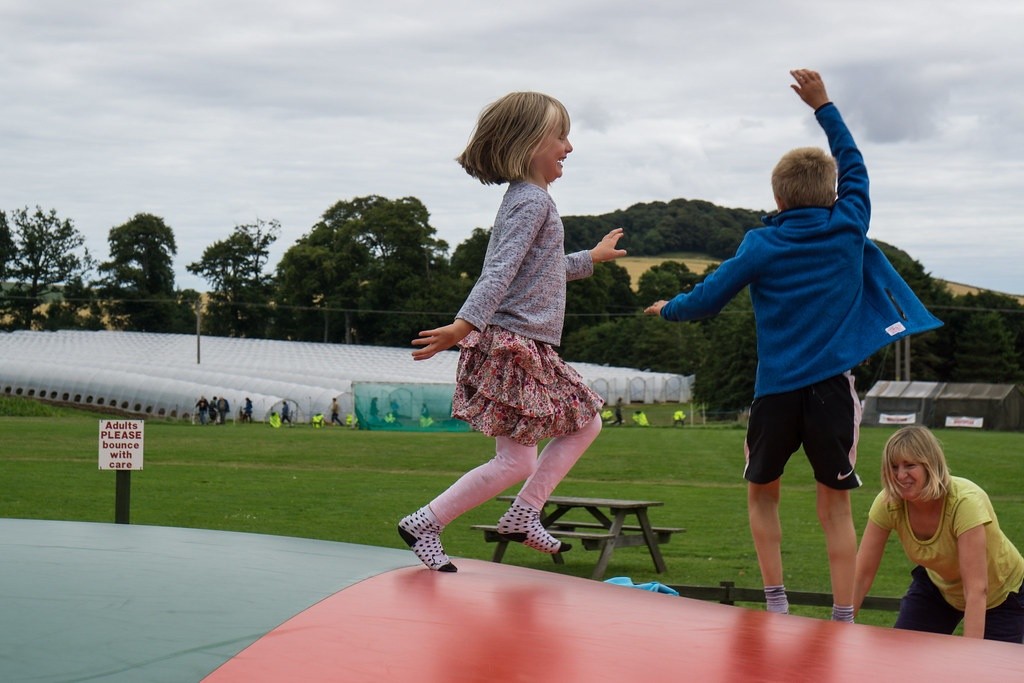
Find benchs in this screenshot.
[467,523,616,549]
[549,520,688,535]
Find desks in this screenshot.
[490,493,669,580]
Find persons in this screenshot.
[421,403,430,419]
[279,401,292,423]
[389,399,400,418]
[195,395,210,425]
[217,396,230,424]
[847,424,1024,645]
[241,397,253,424]
[208,395,220,425]
[369,397,380,419]
[330,398,344,427]
[643,68,945,624]
[611,396,626,426]
[398,92,627,572]
